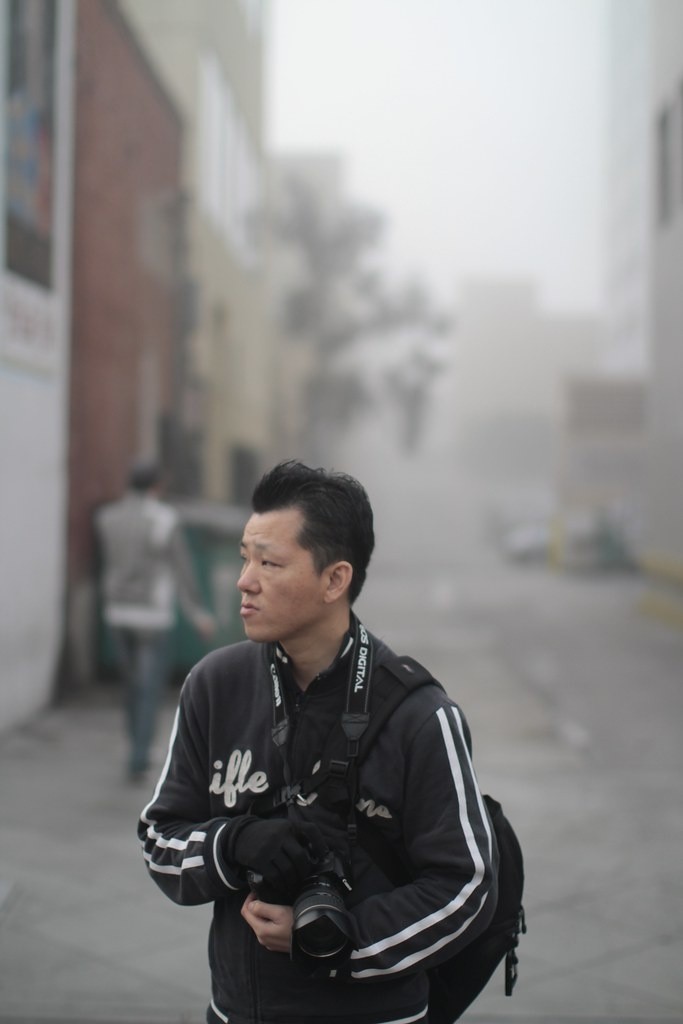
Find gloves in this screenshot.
[234,817,324,892]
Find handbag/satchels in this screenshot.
[428,794,525,1024]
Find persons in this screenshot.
[136,462,524,1023]
[94,462,221,790]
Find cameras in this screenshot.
[247,843,363,978]
